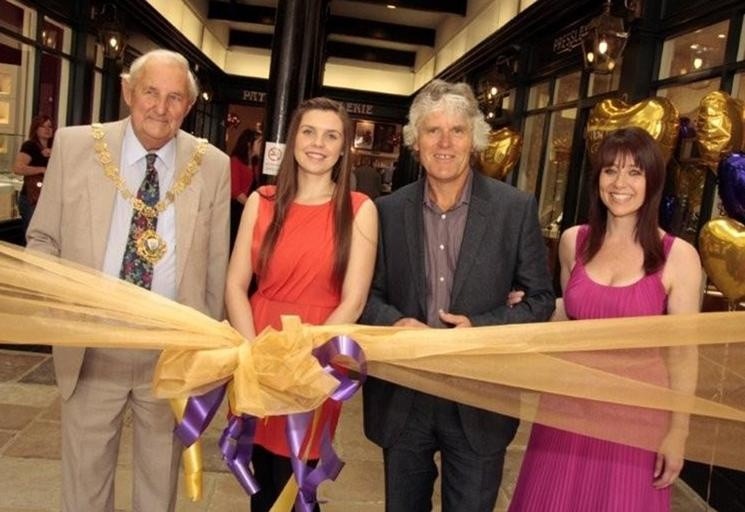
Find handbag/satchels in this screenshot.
[24,174,43,206]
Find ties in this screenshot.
[118,153,160,290]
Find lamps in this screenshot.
[91,0,131,70]
[479,53,520,118]
[577,0,645,77]
